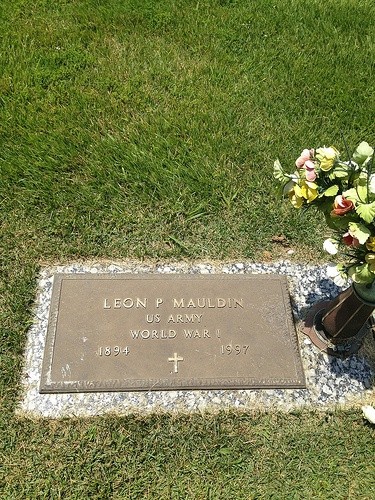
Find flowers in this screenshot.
[272,140,374,305]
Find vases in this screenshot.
[321,282,375,342]
